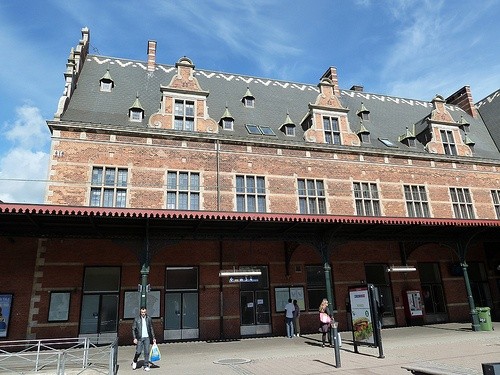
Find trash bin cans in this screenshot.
[475,307,493,331]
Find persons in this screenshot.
[132,306,156,371]
[284,298,295,338]
[319,299,335,348]
[293,300,300,336]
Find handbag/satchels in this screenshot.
[149,342,161,362]
[320,312,333,323]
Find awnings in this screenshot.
[0,202,500,280]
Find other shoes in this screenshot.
[142,366,151,371]
[322,345,325,348]
[297,334,299,337]
[132,361,137,370]
[330,345,332,347]
